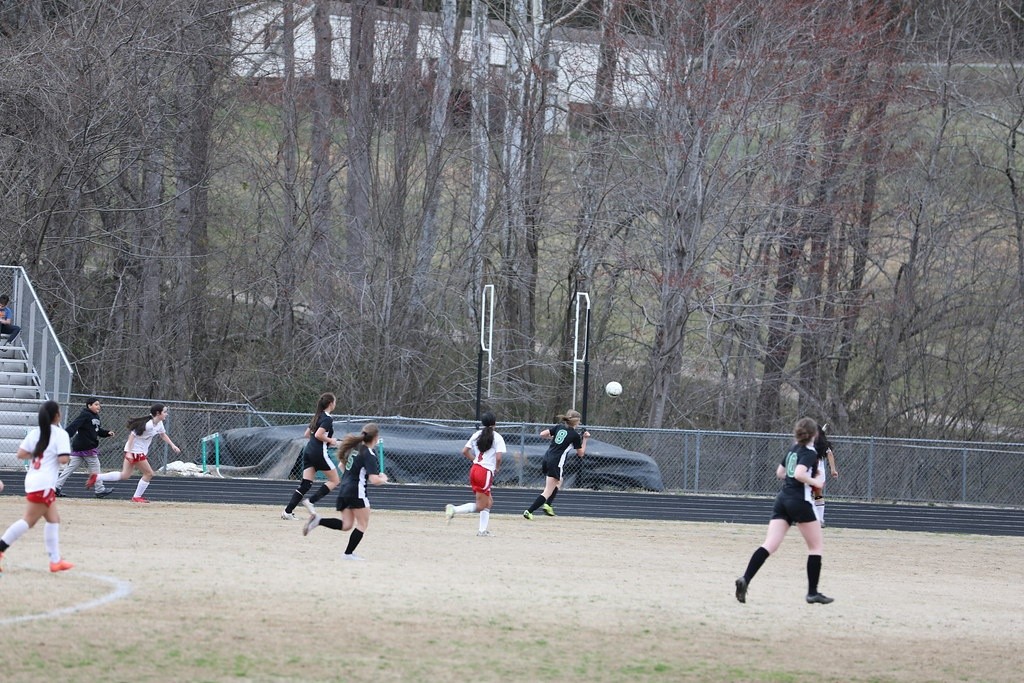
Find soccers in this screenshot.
[606,381,622,399]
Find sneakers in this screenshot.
[302,498,316,515]
[55,488,66,497]
[343,552,355,558]
[524,510,534,520]
[86,472,97,489]
[96,487,115,498]
[806,593,834,604]
[445,504,455,526]
[50,558,73,572]
[736,577,749,603]
[132,495,150,503]
[542,503,555,516]
[281,510,298,521]
[303,514,320,536]
[476,531,496,537]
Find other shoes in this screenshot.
[820,521,826,528]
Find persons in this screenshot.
[0,293,22,353]
[302,422,388,562]
[524,409,591,521]
[85,403,182,503]
[0,401,74,576]
[734,417,841,604]
[444,412,507,538]
[54,398,114,497]
[281,392,340,522]
